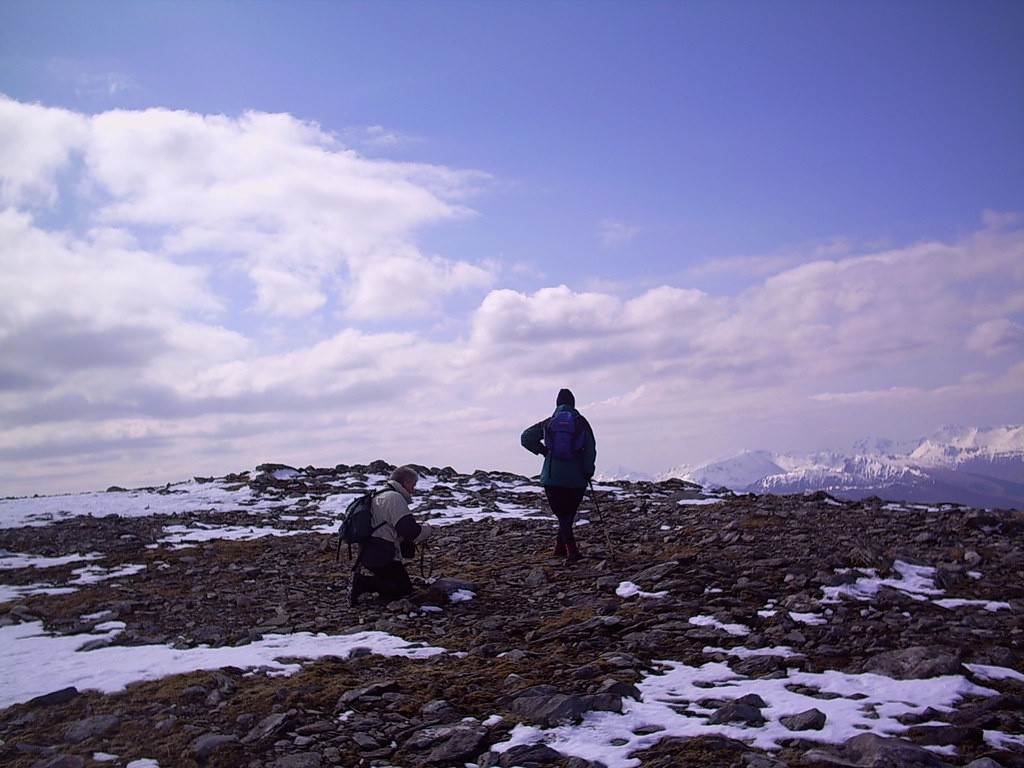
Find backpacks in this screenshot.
[544,412,587,486]
[336,488,397,561]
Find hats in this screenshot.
[557,389,575,408]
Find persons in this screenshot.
[347,466,435,607]
[522,389,597,562]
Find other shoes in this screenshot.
[565,545,582,561]
[347,570,373,609]
[553,540,566,556]
